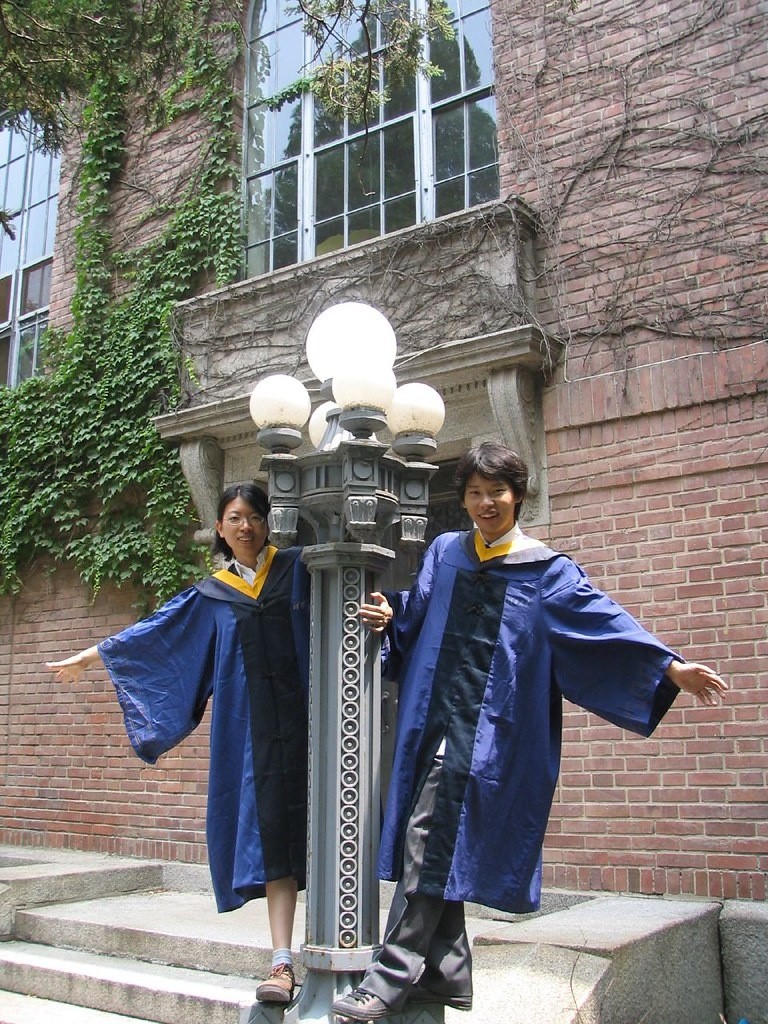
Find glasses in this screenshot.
[221,515,266,526]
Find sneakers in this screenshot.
[255,964,296,1003]
[333,987,472,1022]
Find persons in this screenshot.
[44,483,395,1003]
[330,441,729,1020]
